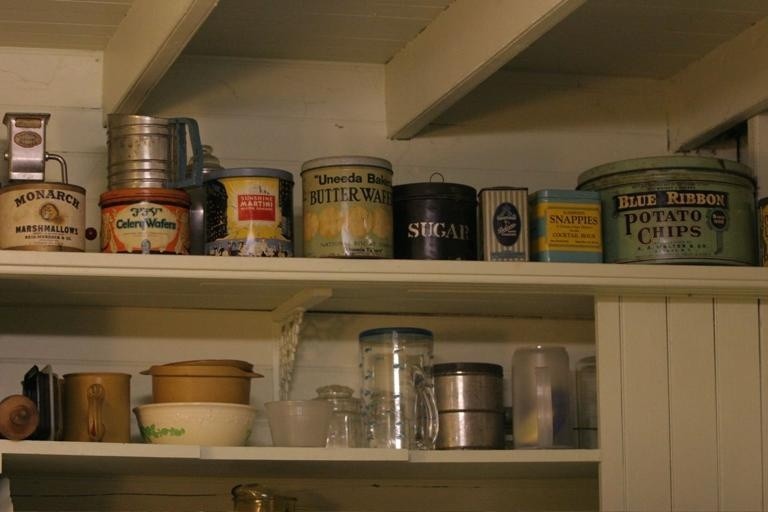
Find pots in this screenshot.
[137,352,265,403]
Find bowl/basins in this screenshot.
[133,401,261,446]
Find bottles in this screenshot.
[357,326,438,451]
[431,359,506,452]
[316,386,364,448]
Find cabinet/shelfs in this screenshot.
[0,247,764,509]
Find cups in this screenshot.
[62,370,132,442]
[510,341,578,451]
[262,399,335,448]
